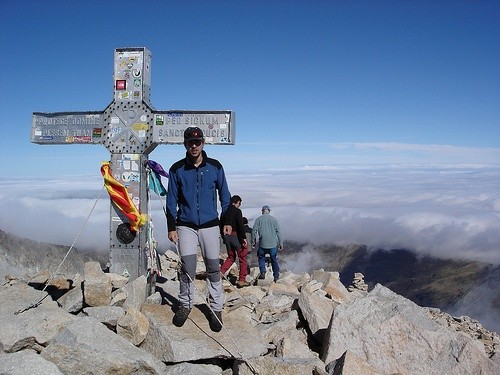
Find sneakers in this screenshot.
[209,312,222,332]
[173,307,189,327]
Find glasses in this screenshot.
[187,141,201,146]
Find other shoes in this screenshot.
[259,273,265,278]
[238,281,250,288]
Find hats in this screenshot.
[262,206,270,211]
[183,127,203,141]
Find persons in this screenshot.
[166,126,234,332]
[251,205,284,282]
[236,217,256,275]
[219,195,251,288]
